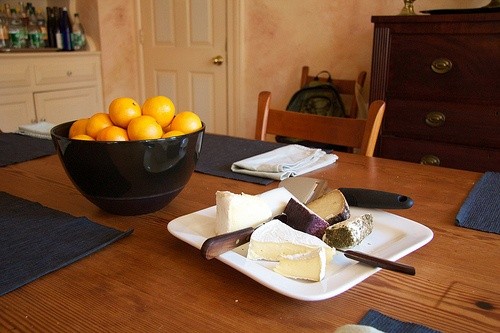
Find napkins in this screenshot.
[18,120,55,140]
[231,143,339,180]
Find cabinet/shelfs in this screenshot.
[0,52,104,133]
[368,14,500,172]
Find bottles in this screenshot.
[0,1,86,53]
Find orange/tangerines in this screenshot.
[68,96,203,141]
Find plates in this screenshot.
[167,203,434,301]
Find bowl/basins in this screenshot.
[50,119,206,216]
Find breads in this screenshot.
[214,187,373,280]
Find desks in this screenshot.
[0,130,500,333]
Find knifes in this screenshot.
[200,214,285,260]
[330,244,416,276]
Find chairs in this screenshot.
[254,91,387,156]
[301,65,367,153]
